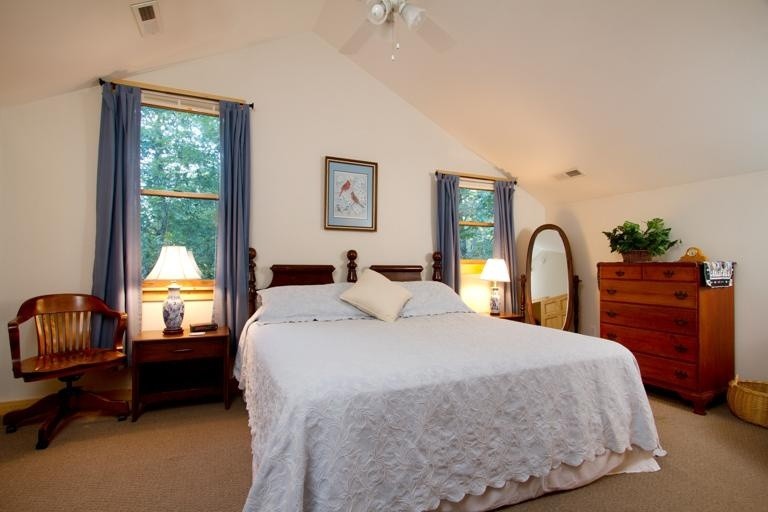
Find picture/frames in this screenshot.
[324,155,378,232]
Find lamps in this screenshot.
[476,258,513,317]
[141,244,206,338]
[361,1,393,34]
[395,0,427,35]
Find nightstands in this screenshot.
[476,312,523,321]
[131,326,234,413]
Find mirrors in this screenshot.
[525,223,581,335]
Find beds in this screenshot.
[238,246,667,512]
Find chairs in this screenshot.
[7,293,129,449]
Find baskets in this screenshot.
[725,373,768,429]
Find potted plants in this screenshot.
[604,218,682,261]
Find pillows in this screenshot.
[337,265,414,326]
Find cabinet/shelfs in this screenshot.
[540,296,572,326]
[596,263,735,415]
